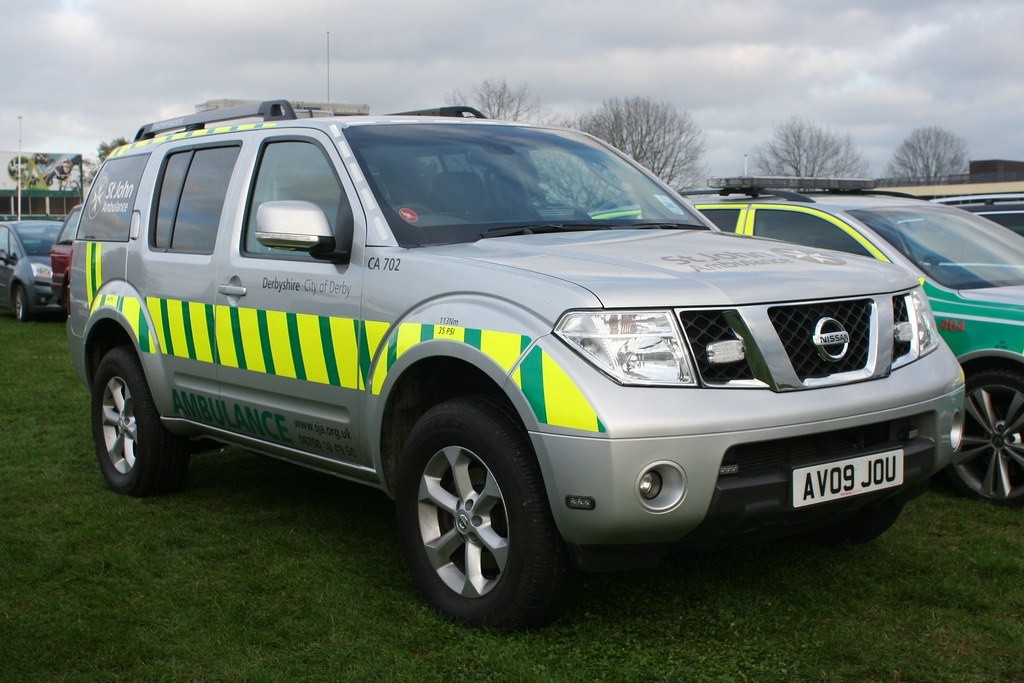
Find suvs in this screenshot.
[579,173,1024,509]
[51,202,82,323]
[61,96,967,631]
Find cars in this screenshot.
[0,220,69,324]
